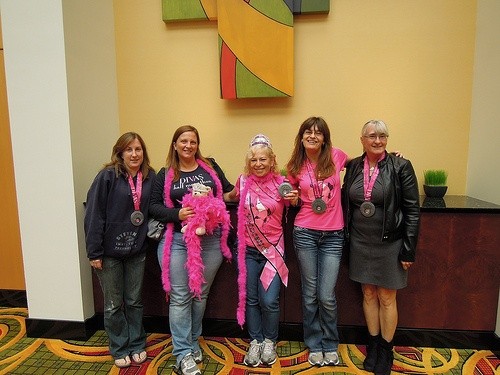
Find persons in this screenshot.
[223,134,299,366]
[287,117,404,366]
[150,126,234,375]
[84,131,154,366]
[341,121,422,375]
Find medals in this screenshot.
[311,198,326,213]
[278,183,292,198]
[360,202,375,216]
[130,211,143,225]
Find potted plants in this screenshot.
[423,170,449,200]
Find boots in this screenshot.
[363,333,381,372]
[374,335,394,375]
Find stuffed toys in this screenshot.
[180,184,211,235]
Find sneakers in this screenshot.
[192,349,202,364]
[324,351,339,365]
[181,354,201,375]
[308,351,324,366]
[244,339,264,367]
[260,338,277,365]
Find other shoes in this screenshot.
[115,355,131,368]
[132,351,147,364]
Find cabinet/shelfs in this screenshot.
[82,195,500,353]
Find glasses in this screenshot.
[362,133,388,140]
[304,129,323,136]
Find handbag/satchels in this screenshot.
[147,165,168,241]
